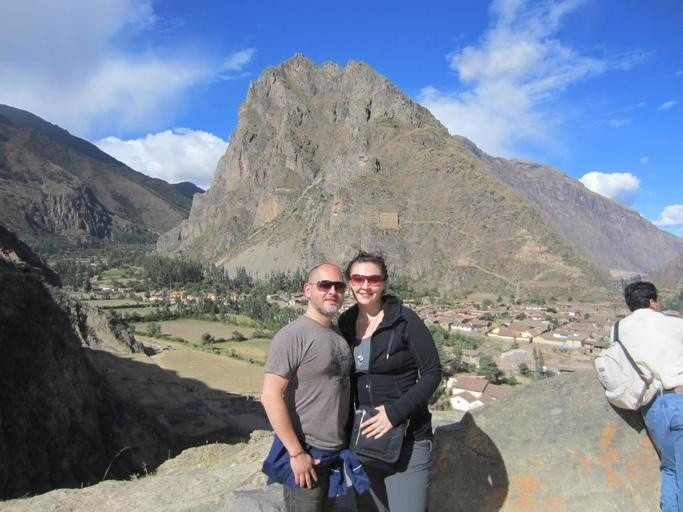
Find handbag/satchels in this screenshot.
[348,405,411,471]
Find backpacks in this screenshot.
[593,321,663,411]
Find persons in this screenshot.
[609,282,683,512]
[338,251,441,511]
[260,262,359,511]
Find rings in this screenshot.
[379,424,384,430]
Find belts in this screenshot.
[656,389,674,394]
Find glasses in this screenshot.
[351,274,383,286]
[308,280,347,293]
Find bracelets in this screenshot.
[290,449,304,460]
